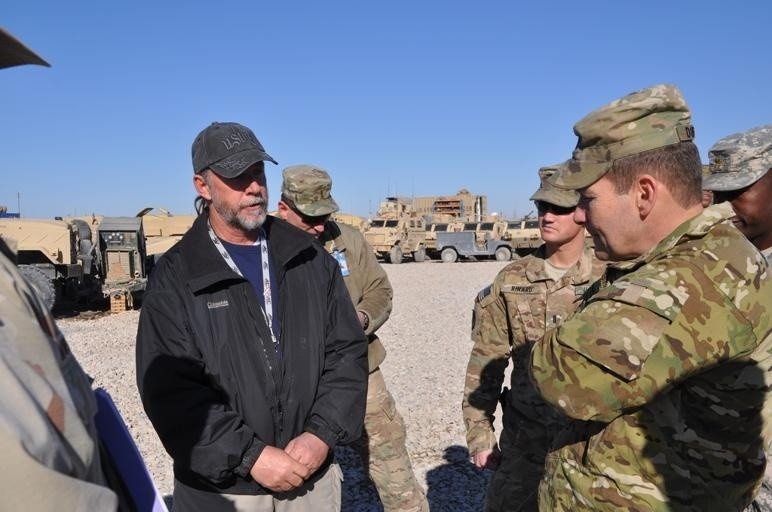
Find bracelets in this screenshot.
[363,313,368,328]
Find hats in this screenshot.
[696,125,772,193]
[1,22,50,74]
[543,82,698,191]
[279,166,340,220]
[527,163,587,212]
[187,120,280,180]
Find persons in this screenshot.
[462,162,610,512]
[136,121,369,511]
[528,84,772,512]
[1,246,117,512]
[702,126,771,273]
[279,164,430,512]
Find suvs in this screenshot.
[505,211,544,249]
[426,223,464,250]
[462,219,505,247]
[437,232,513,262]
[362,217,426,263]
[0,217,93,314]
